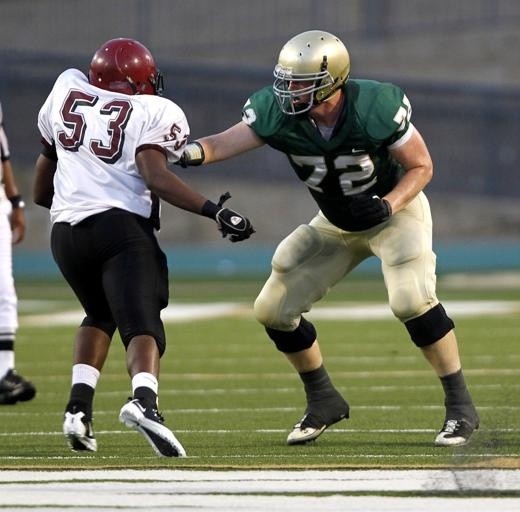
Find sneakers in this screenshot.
[286,395,350,445]
[63,400,98,454]
[118,397,187,458]
[0,368,35,405]
[434,404,480,447]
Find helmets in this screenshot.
[273,30,351,115]
[88,37,164,95]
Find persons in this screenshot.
[31,36,256,457]
[0,105,36,407]
[173,29,480,448]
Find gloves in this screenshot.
[349,194,389,230]
[212,191,256,242]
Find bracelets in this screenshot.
[8,192,25,209]
